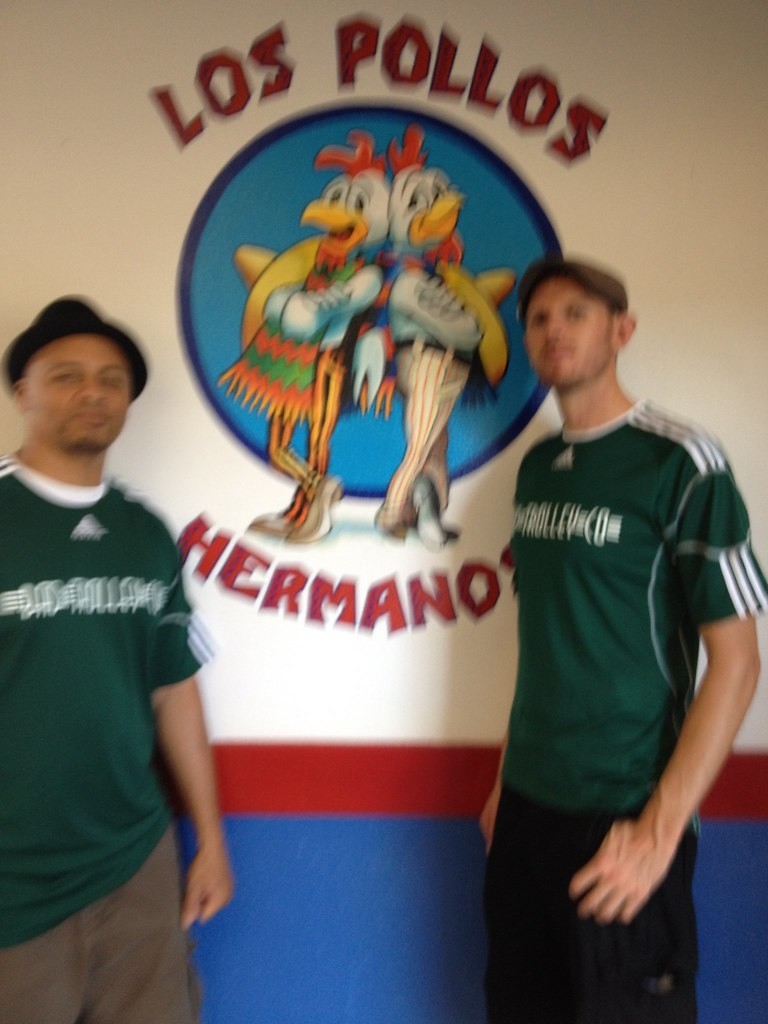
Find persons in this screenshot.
[0,294,235,1023]
[476,260,768,1024]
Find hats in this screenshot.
[517,258,627,319]
[5,298,148,407]
